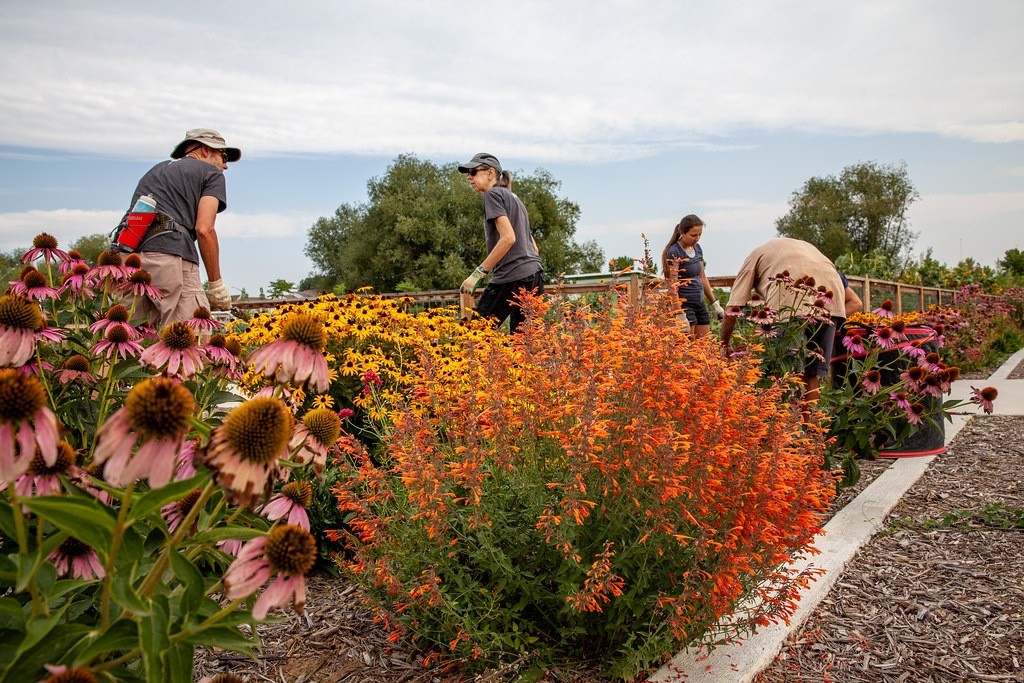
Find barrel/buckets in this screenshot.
[831,325,946,457]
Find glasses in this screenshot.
[220,149,229,162]
[468,167,489,177]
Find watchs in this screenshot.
[478,265,488,275]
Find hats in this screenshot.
[458,153,503,175]
[170,128,241,162]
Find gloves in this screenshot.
[206,279,232,311]
[460,268,486,297]
[712,300,724,320]
[675,313,691,332]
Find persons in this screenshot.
[662,214,863,426]
[108,128,242,335]
[458,153,544,336]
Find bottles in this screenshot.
[116,194,157,252]
